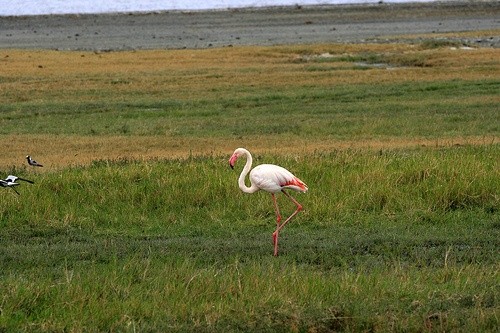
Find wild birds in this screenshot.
[0,174,34,195]
[25,155,43,170]
[228,148,308,257]
